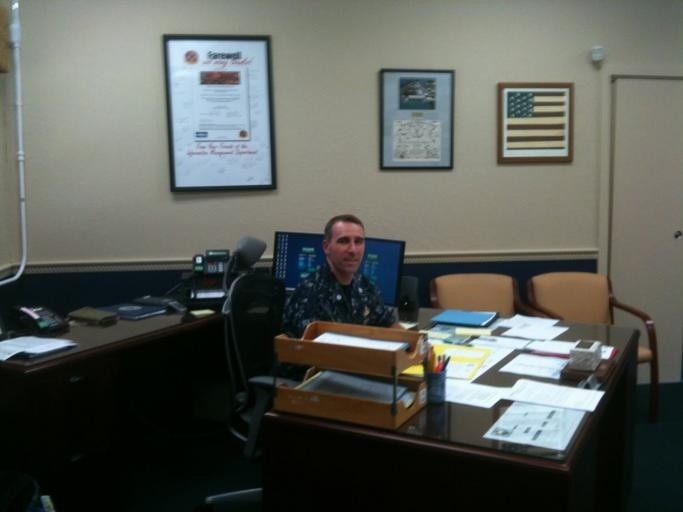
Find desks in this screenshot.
[262,305,639,510]
[0,304,229,468]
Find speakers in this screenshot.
[397,273,419,321]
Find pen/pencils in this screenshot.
[425,346,452,372]
[530,351,569,359]
[473,335,498,341]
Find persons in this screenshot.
[280,214,436,381]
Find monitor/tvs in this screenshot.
[272,231,406,306]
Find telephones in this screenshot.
[8,303,69,334]
[192,249,231,278]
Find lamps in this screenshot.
[218,236,265,290]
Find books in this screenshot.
[431,309,499,327]
[68,305,116,324]
[103,303,166,321]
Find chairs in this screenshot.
[430,271,657,423]
[202,273,301,506]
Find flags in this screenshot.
[506,90,567,151]
